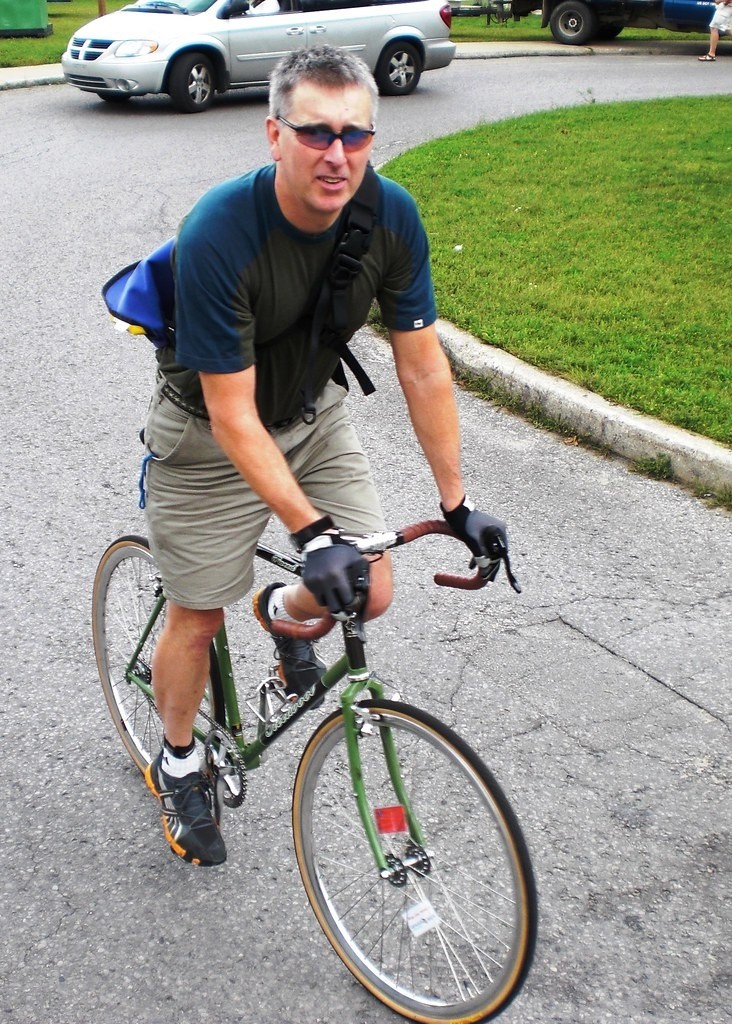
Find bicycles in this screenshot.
[89,506,539,1024]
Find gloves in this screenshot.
[440,492,508,579]
[300,525,370,620]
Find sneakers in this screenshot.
[252,582,324,709]
[145,749,227,866]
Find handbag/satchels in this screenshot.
[102,236,177,350]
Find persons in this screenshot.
[698,0,732,61]
[139,45,508,867]
[242,0,280,15]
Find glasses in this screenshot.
[276,116,376,152]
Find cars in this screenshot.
[507,0,729,47]
[61,0,458,112]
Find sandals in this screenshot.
[698,53,716,61]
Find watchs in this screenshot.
[289,514,334,553]
